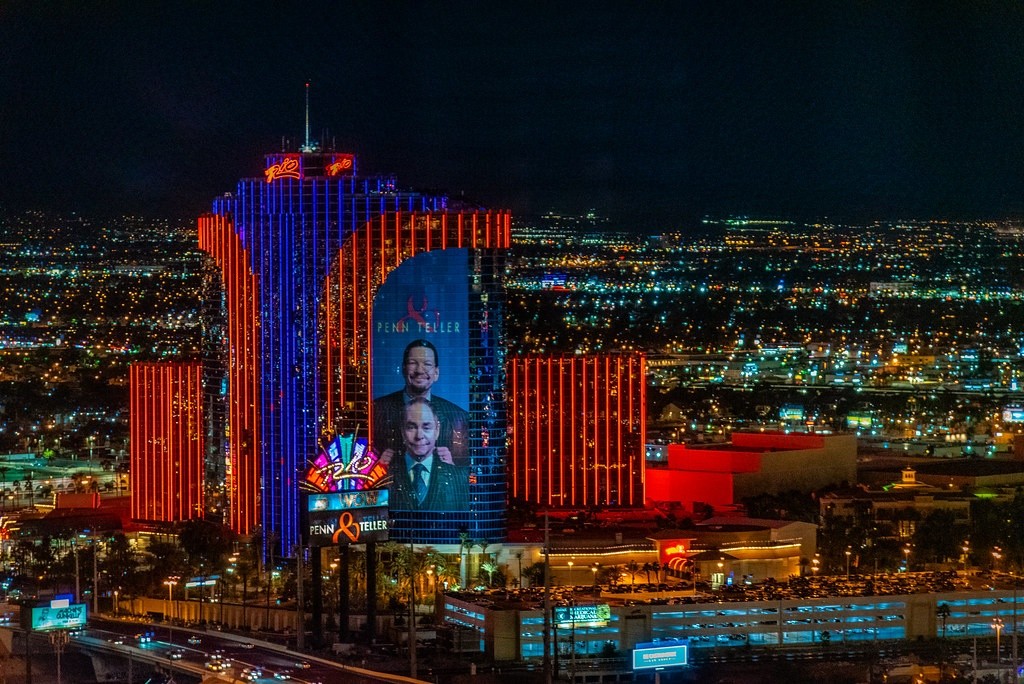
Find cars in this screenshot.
[188,636,202,645]
[134,633,151,643]
[166,650,182,660]
[240,667,264,683]
[238,642,255,650]
[273,670,291,681]
[204,651,232,672]
[471,598,495,606]
[294,660,311,670]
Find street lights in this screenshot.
[993,616,1004,668]
[959,540,973,575]
[844,544,855,581]
[991,545,1003,571]
[205,575,222,629]
[567,557,576,586]
[591,561,600,594]
[902,541,916,577]
[811,552,822,575]
[163,575,182,624]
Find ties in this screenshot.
[411,464,430,504]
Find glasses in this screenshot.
[405,360,435,372]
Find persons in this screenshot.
[373,338,469,465]
[374,396,469,512]
[36,608,52,626]
